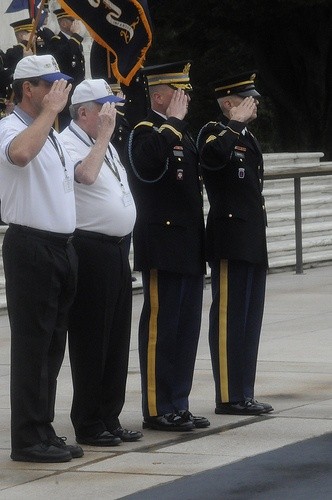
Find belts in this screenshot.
[76,229,129,244]
[8,224,74,245]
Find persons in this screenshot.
[123,58,211,432]
[59,78,143,447]
[0,55,77,463]
[196,72,274,415]
[2,18,44,105]
[104,78,144,272]
[48,9,85,133]
[90,39,154,123]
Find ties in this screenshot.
[246,130,252,143]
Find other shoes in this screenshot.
[215,399,267,415]
[109,425,143,442]
[142,413,195,431]
[44,435,84,459]
[9,443,72,463]
[244,397,274,412]
[173,410,211,428]
[76,430,122,446]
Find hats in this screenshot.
[209,69,262,99]
[10,17,38,34]
[13,54,72,83]
[71,78,128,105]
[140,60,194,93]
[53,8,76,21]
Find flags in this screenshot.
[56,0,152,86]
